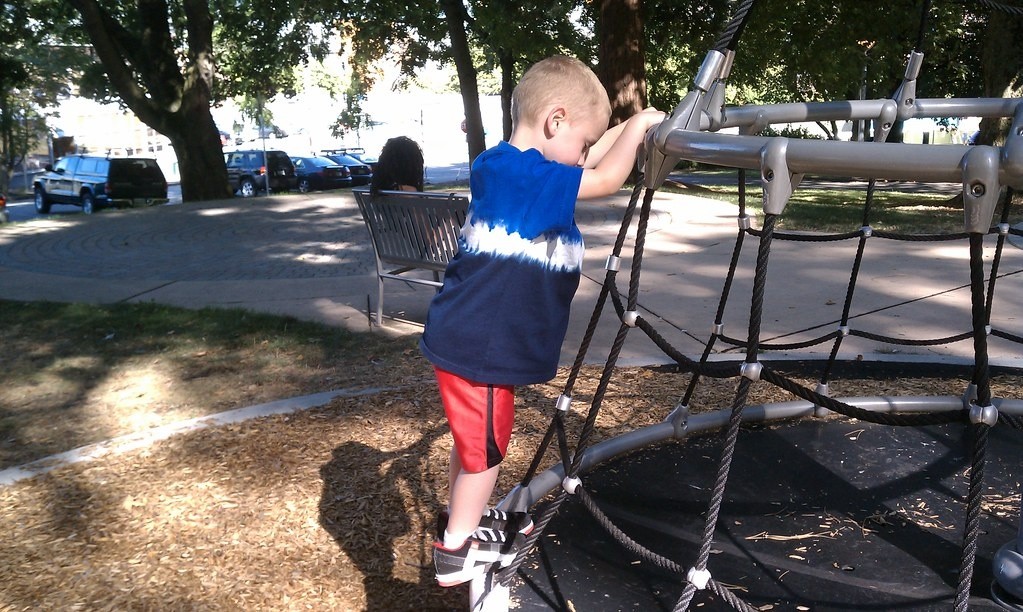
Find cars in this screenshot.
[288,156,353,194]
[326,153,373,186]
[352,152,379,172]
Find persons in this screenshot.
[417,55,670,589]
[370,136,467,261]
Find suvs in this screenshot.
[223,150,298,197]
[29,156,172,215]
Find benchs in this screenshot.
[352,188,469,327]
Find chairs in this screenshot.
[234,156,257,165]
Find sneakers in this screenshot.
[431,528,527,587]
[437,508,534,535]
